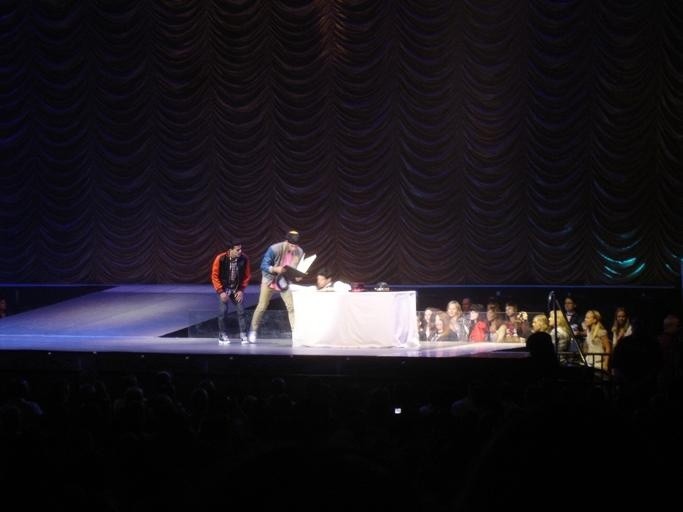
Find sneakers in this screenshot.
[218,331,232,344]
[239,332,258,345]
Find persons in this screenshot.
[316,272,350,291]
[249,230,305,343]
[211,239,251,344]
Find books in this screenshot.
[282,253,318,282]
